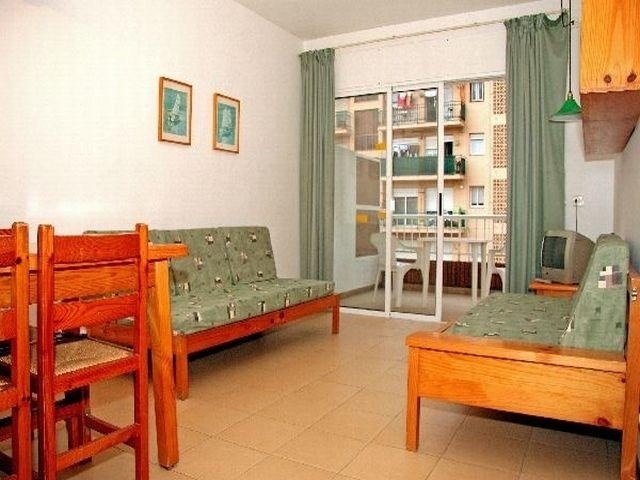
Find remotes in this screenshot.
[534,278,552,285]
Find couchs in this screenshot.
[81,224,340,401]
[403,232,640,479]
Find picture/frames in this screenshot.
[211,91,243,155]
[155,73,196,150]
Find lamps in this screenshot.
[546,0,583,125]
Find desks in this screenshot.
[414,234,493,304]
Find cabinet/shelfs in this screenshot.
[575,0,639,165]
[527,277,583,298]
[330,146,383,297]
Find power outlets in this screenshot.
[571,196,585,210]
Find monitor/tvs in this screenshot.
[540,229,596,285]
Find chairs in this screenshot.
[369,231,426,306]
[482,243,506,297]
[2,214,150,479]
